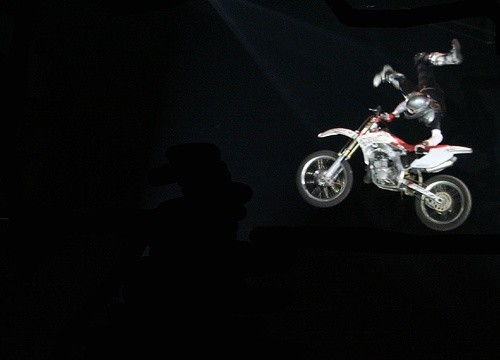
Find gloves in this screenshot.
[415,143,429,155]
[384,112,393,123]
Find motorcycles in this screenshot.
[294,103,475,233]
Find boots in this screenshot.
[373,64,406,91]
[429,38,463,67]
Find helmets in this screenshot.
[404,92,432,120]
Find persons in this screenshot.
[373,38,463,153]
